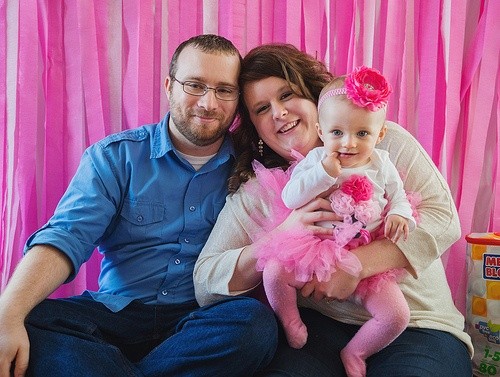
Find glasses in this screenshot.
[169,76,240,100]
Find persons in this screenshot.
[243,65,423,377]
[193,43,475,377]
[0,36,281,377]
